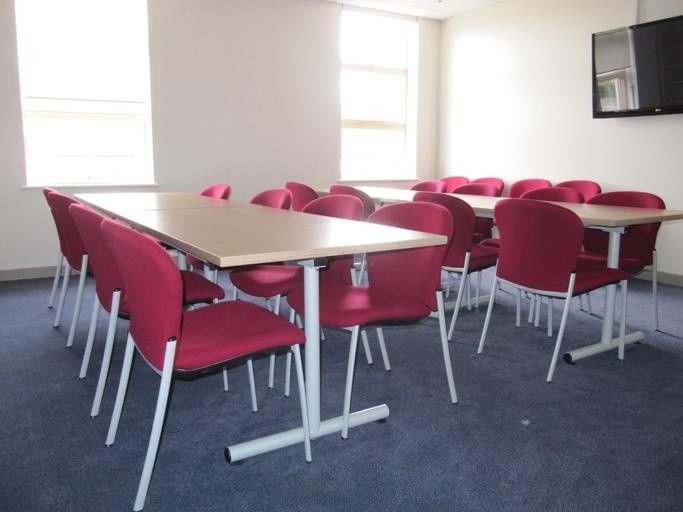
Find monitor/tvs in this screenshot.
[591,15,683,118]
